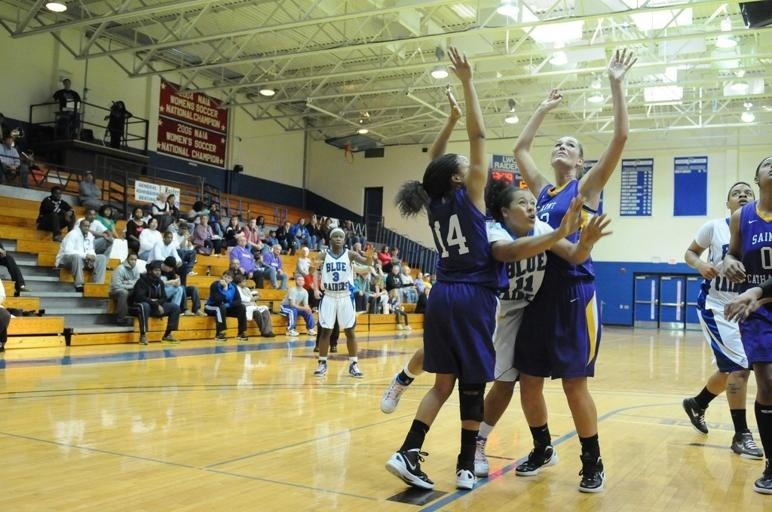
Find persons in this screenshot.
[381,184,613,477]
[517,47,640,493]
[723,156,772,493]
[51,78,81,112]
[1,132,433,355]
[722,276,772,321]
[680,182,766,459]
[382,44,500,491]
[314,228,375,376]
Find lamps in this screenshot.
[716,36,738,49]
[358,129,368,134]
[429,68,448,80]
[730,78,749,93]
[504,109,520,125]
[259,89,275,97]
[548,50,569,66]
[741,111,755,122]
[587,93,603,104]
[495,2,518,17]
[45,0,67,13]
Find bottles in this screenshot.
[206,266,212,276]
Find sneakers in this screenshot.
[161,333,180,344]
[137,335,149,346]
[379,373,406,415]
[454,452,476,492]
[215,332,228,342]
[306,328,318,337]
[576,453,607,494]
[514,440,559,479]
[474,438,490,479]
[285,328,301,337]
[393,323,412,332]
[313,359,329,378]
[727,430,764,462]
[235,332,249,341]
[384,447,437,494]
[753,458,772,496]
[681,396,711,437]
[346,360,365,381]
[329,345,339,353]
